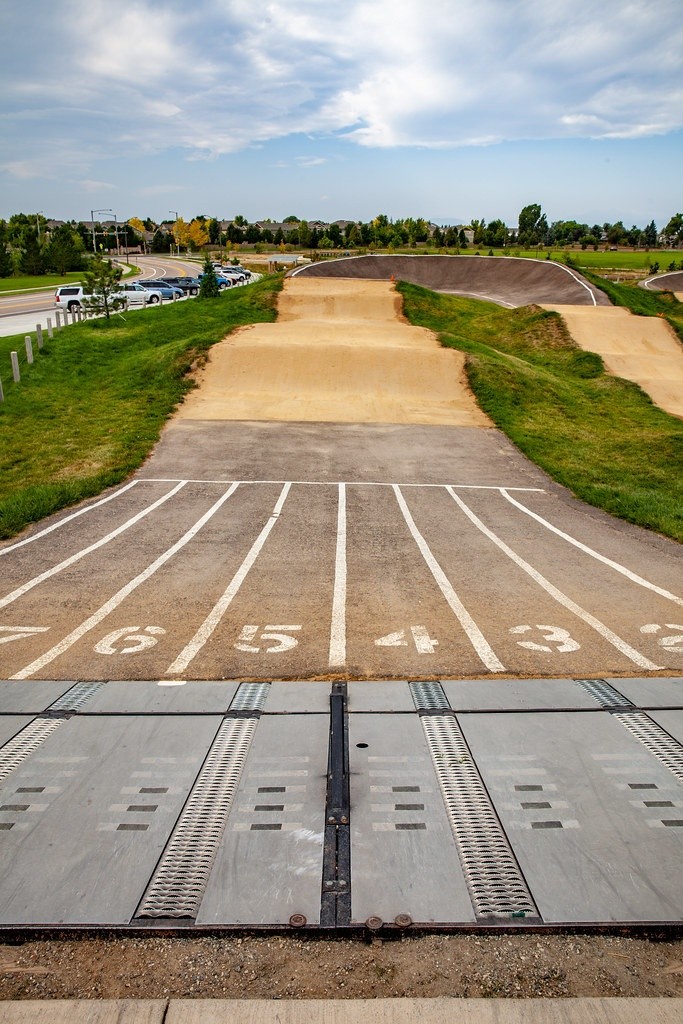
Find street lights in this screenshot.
[98,212,119,256]
[90,208,113,253]
[169,210,179,256]
[36,210,43,234]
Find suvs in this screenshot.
[138,279,184,299]
[54,286,130,312]
[112,281,163,304]
[156,262,252,295]
